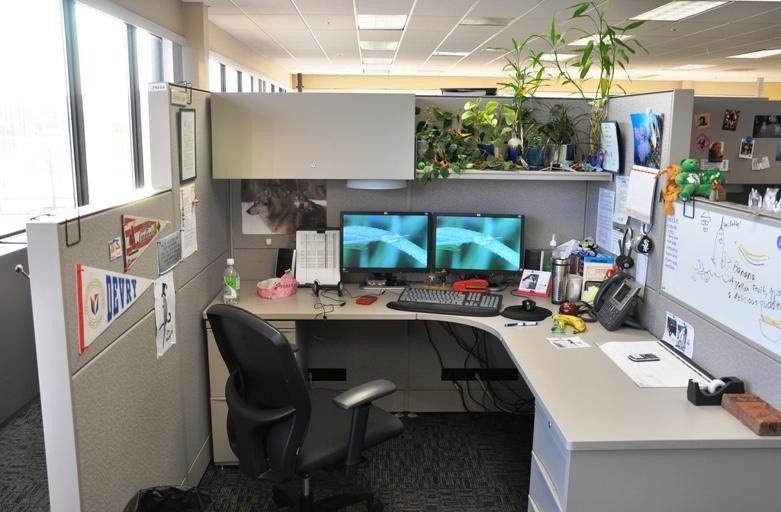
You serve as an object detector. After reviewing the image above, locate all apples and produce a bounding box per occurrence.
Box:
[560,302,576,315]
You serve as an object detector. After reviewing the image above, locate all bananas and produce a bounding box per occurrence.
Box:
[553,314,585,333]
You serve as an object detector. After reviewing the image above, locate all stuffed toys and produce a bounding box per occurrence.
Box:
[659,158,729,218]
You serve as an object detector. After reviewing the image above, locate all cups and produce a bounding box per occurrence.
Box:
[552,257,582,304]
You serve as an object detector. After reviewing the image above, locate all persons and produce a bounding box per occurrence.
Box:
[754,116,781,138]
[709,142,724,161]
[676,327,687,348]
[526,274,536,289]
[725,111,738,131]
[644,120,662,168]
[698,115,709,127]
[743,142,754,157]
[696,136,707,147]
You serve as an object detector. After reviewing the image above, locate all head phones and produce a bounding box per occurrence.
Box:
[616,228,634,269]
[313,280,343,297]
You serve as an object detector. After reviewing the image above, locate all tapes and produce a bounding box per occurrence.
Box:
[707,379,726,394]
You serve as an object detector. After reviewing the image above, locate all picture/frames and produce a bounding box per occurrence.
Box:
[178,108,197,182]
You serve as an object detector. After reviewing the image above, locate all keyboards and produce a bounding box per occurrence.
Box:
[388,287,504,316]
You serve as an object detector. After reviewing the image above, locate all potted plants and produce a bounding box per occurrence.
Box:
[460,99,506,168]
[478,2,649,167]
[414,107,454,166]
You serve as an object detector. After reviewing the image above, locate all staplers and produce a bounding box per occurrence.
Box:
[452,279,490,293]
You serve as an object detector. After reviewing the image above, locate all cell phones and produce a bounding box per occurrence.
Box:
[356,295,377,304]
[629,353,658,362]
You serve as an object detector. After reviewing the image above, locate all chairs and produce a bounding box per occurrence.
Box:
[206,303,404,508]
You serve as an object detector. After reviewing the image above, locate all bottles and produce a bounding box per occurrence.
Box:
[223,258,240,306]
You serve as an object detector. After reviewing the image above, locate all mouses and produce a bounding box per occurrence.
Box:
[523,300,536,311]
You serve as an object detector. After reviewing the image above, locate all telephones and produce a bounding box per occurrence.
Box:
[592,274,640,331]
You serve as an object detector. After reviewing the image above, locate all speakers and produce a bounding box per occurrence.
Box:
[275,247,296,279]
[523,249,543,271]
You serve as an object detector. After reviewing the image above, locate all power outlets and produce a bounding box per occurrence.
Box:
[441,368,518,381]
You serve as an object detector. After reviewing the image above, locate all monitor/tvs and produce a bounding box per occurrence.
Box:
[340,211,433,290]
[432,213,525,291]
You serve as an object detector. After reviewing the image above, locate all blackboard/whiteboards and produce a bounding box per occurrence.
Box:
[659,199,781,363]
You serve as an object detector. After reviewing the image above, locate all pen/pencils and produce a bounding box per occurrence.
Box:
[504,321,537,326]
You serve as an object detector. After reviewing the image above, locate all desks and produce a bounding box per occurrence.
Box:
[204,280,779,512]
